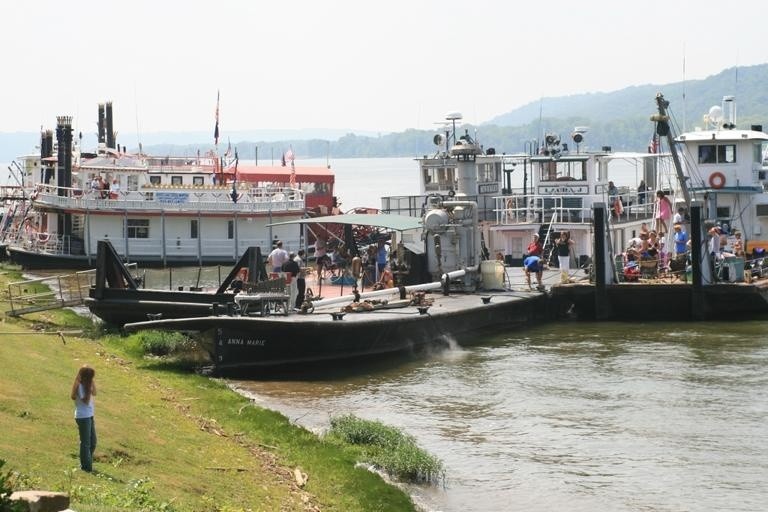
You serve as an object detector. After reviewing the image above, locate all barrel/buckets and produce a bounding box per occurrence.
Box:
[505,254,513,264]
[579,254,589,267]
[480,259,507,292]
[723,256,745,283]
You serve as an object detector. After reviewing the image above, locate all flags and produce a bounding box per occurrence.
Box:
[284,148,293,161]
[226,143,232,158]
[650,122,660,155]
[289,161,296,188]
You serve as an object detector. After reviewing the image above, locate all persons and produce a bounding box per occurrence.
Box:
[556,231,574,281]
[636,181,648,203]
[608,182,623,224]
[655,190,673,234]
[71,365,97,472]
[528,235,544,285]
[266,231,387,308]
[624,208,746,280]
[524,256,547,291]
[72,171,119,201]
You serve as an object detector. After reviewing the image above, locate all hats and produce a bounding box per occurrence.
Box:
[288,251,297,258]
[531,232,541,238]
[673,224,682,230]
[708,225,718,234]
